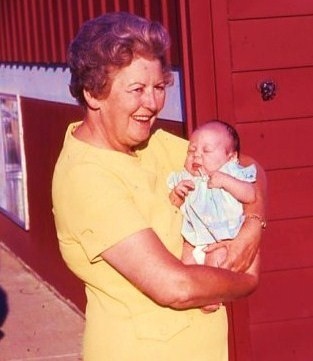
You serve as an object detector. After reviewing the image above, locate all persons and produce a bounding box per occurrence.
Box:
[164,119,257,314]
[51,12,266,361]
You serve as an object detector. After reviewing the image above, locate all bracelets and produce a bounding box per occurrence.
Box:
[241,214,266,234]
